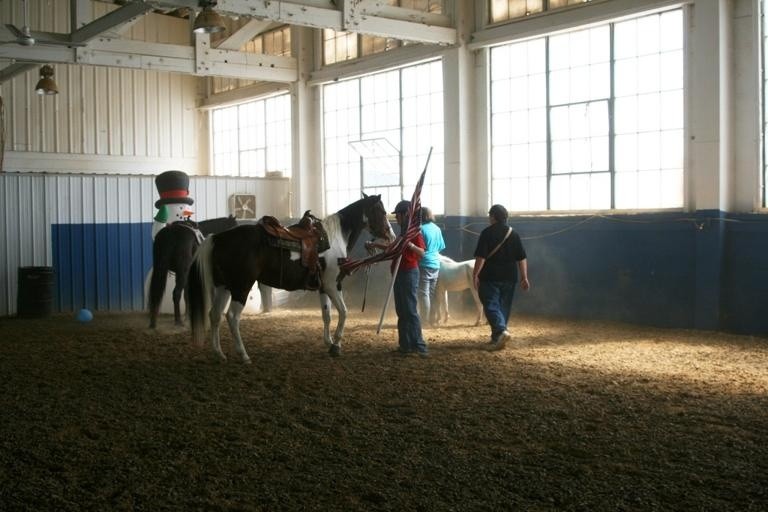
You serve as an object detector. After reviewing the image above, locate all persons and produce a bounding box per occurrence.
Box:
[471,204,532,351]
[364,200,432,357]
[415,206,446,330]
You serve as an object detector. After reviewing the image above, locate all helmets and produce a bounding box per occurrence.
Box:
[391,201,411,214]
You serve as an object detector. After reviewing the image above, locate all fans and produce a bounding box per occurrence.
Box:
[232,194,256,220]
[0,1,88,49]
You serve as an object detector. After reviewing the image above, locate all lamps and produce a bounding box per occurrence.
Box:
[36,65,59,96]
[191,0,226,35]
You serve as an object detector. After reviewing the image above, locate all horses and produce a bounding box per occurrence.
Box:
[435,253,489,326]
[146,213,239,329]
[185,190,397,365]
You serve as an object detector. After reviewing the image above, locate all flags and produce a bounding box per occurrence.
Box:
[337,167,429,276]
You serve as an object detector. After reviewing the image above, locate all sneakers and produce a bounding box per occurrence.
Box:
[486,330,511,350]
[394,349,429,358]
